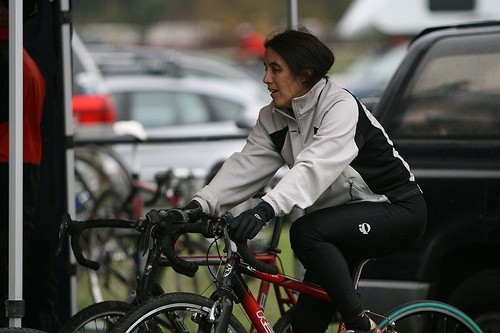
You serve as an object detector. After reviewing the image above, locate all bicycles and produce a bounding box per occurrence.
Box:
[62,135,262,326]
[55,212,480,333]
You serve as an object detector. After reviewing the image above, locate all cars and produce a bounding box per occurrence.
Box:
[86,46,273,195]
[368,19,500,299]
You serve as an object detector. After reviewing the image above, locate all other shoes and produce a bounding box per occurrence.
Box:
[340,310,381,333]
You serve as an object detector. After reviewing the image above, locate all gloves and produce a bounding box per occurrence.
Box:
[146,200,203,223]
[230,200,275,241]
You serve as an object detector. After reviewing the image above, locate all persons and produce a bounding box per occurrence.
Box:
[0,0,45,329]
[163,30,428,333]
